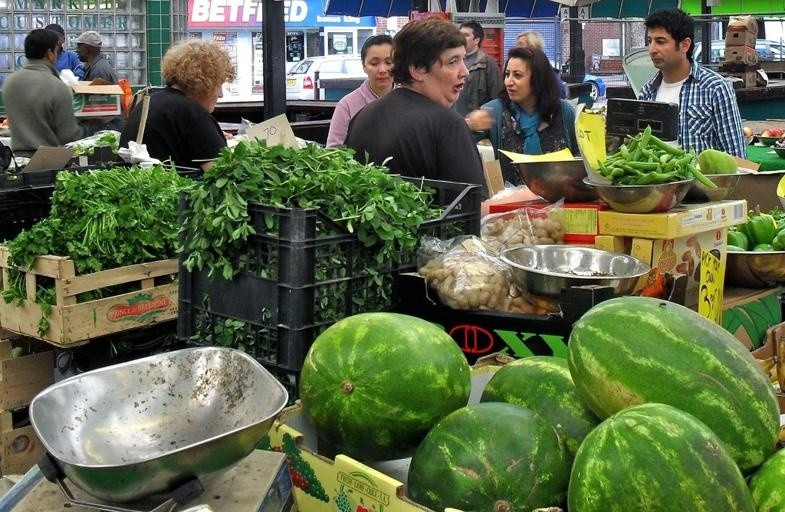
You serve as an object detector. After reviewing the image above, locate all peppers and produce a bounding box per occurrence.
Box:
[592,124,718,190]
[727,203,785,252]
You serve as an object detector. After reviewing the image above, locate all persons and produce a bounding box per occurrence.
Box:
[115,38,235,180]
[344,17,541,218]
[325,34,402,149]
[1,28,91,159]
[514,32,566,100]
[448,19,503,118]
[637,7,746,166]
[70,31,117,85]
[45,23,85,79]
[461,47,580,158]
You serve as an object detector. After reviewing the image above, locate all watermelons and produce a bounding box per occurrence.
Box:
[743,451,785,511]
[566,399,758,511]
[408,402,564,512]
[479,355,596,468]
[300,312,471,458]
[566,295,783,473]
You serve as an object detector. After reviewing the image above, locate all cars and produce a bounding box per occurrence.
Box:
[550,59,606,102]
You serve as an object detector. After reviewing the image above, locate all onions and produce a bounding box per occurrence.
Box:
[743,127,785,143]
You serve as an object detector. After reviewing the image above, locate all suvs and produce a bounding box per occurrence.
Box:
[286,51,368,102]
[689,38,785,63]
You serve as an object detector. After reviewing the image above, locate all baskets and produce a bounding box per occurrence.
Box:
[0,164,200,246]
[177,172,482,372]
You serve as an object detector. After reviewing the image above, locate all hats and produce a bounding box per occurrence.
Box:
[71,30,102,47]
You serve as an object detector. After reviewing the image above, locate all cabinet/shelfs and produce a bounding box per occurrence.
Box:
[0,0,189,116]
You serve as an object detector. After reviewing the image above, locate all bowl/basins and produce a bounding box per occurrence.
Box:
[502,242,656,304]
[725,251,785,289]
[743,134,785,159]
[508,156,751,214]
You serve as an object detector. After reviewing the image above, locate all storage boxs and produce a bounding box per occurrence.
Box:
[716,72,757,87]
[725,30,756,48]
[727,16,761,33]
[70,78,125,123]
[724,45,758,66]
[255,351,515,512]
[686,285,784,352]
